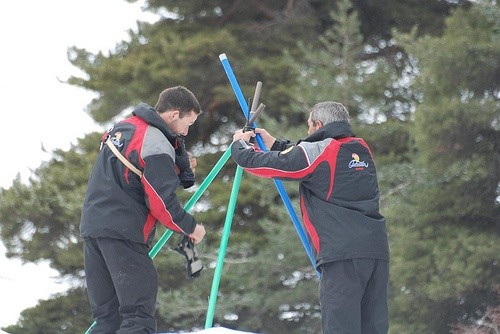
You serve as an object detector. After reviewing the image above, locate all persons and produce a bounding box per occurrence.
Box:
[230,102,389,334]
[80,86,205,334]
